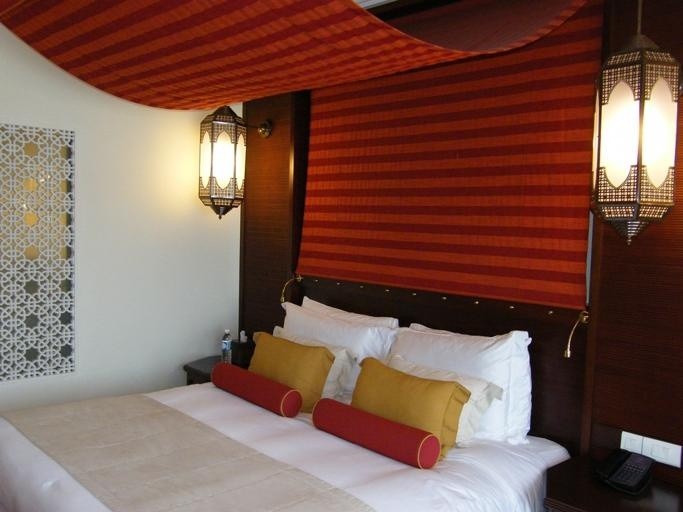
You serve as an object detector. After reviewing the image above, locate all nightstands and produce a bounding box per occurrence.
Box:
[543,449,683,512]
[182,339,255,385]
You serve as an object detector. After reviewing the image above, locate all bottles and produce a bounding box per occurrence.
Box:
[221,329,232,365]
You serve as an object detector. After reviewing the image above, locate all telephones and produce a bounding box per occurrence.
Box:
[599,448,656,492]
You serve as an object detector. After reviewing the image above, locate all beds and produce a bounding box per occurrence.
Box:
[1,276,592,511]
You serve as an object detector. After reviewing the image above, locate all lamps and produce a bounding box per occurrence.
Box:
[592,1,679,245]
[199,105,271,220]
